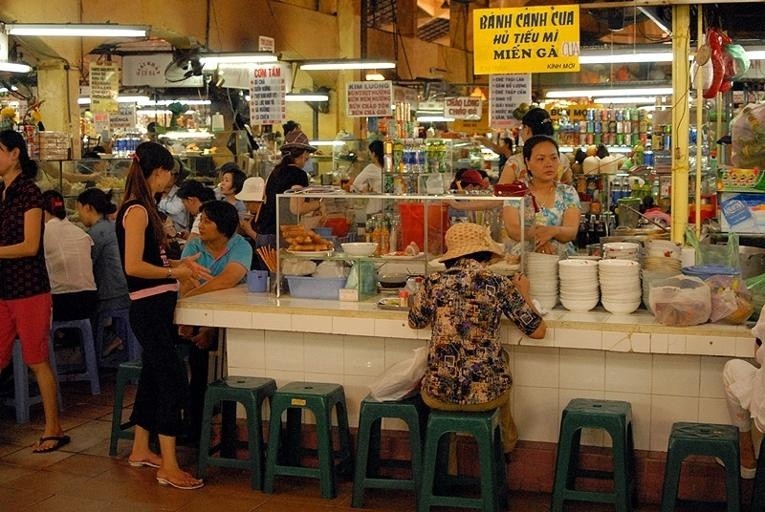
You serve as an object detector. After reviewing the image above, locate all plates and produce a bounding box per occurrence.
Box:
[172,154,181,159]
[96,153,116,159]
[183,151,203,156]
[381,251,425,259]
[596,259,643,315]
[286,248,334,255]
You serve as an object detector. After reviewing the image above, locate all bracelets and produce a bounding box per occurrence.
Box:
[164,267,173,279]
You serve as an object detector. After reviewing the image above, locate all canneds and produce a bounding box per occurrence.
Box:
[384,141,393,193]
[579,108,646,145]
[652,125,672,152]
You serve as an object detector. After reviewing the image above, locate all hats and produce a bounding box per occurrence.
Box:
[461,170,482,184]
[235,177,265,202]
[280,127,317,152]
[438,222,504,264]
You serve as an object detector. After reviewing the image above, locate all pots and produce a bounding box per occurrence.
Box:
[694,232,765,281]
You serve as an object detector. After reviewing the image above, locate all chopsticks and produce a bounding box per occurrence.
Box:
[255,245,276,271]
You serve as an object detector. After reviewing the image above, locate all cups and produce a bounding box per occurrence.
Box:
[643,151,653,165]
[269,271,284,295]
[612,189,629,204]
[246,270,268,291]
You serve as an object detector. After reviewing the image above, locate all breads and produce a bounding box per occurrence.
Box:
[280,223,330,250]
[186,143,199,152]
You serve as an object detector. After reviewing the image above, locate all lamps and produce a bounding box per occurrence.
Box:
[414,109,455,123]
[306,139,347,146]
[0,61,33,74]
[544,0,765,103]
[4,22,152,38]
[297,57,397,79]
[241,90,333,107]
[76,89,215,147]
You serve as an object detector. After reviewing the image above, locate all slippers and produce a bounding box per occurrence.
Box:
[716,456,757,479]
[33,435,71,453]
[157,469,204,490]
[128,454,161,468]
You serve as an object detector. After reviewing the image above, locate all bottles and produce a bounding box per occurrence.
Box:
[412,277,422,307]
[711,156,717,168]
[364,211,403,256]
[381,137,447,196]
[399,290,408,308]
[631,178,651,200]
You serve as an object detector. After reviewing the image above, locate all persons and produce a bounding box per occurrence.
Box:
[710,301,765,480]
[153,159,256,425]
[147,122,164,141]
[0,128,73,454]
[241,121,320,276]
[504,136,581,256]
[350,140,383,194]
[497,108,573,190]
[226,121,258,176]
[115,141,206,489]
[77,187,132,312]
[408,219,546,465]
[39,190,99,361]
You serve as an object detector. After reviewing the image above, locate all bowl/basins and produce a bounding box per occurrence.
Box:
[313,226,332,237]
[600,241,642,259]
[324,218,350,237]
[524,251,558,312]
[556,258,601,312]
[340,242,378,257]
[642,238,683,315]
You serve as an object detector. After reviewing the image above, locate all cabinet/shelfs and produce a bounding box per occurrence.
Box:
[653,108,718,200]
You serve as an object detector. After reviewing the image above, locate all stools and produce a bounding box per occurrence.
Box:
[661,422,741,512]
[0,309,140,455]
[351,400,423,507]
[551,397,637,512]
[198,376,277,490]
[261,381,351,500]
[420,407,505,512]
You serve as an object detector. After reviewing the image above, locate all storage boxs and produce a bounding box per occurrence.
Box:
[719,192,765,234]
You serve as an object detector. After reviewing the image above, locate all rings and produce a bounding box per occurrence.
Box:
[542,250,545,254]
[534,239,540,245]
